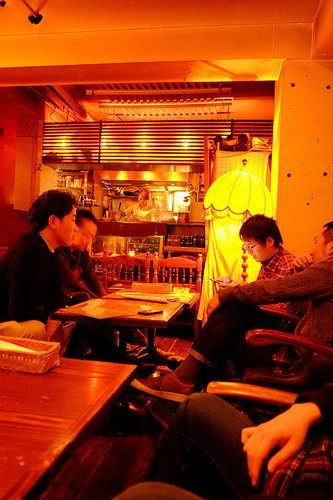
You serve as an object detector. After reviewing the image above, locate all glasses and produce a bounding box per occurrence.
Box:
[244,241,260,251]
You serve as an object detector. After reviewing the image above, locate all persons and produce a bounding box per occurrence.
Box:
[54,208,184,366]
[144,383,333,500]
[129,224,333,402]
[0,189,156,378]
[206,214,308,376]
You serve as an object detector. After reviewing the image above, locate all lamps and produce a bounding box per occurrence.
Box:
[203,160,273,281]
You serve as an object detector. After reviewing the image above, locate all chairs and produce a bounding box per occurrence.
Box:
[1,245,333,416]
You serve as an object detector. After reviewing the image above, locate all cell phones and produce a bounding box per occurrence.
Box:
[138,309,162,315]
[210,278,223,284]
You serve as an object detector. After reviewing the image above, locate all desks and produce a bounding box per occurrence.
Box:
[102,287,200,361]
[51,299,184,364]
[0,357,137,500]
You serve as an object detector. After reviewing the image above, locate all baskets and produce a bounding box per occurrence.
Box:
[0,336,61,374]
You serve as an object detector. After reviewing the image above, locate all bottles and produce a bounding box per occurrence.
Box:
[180,235,205,247]
[104,208,109,218]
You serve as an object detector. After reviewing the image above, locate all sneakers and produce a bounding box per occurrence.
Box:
[130,370,194,403]
